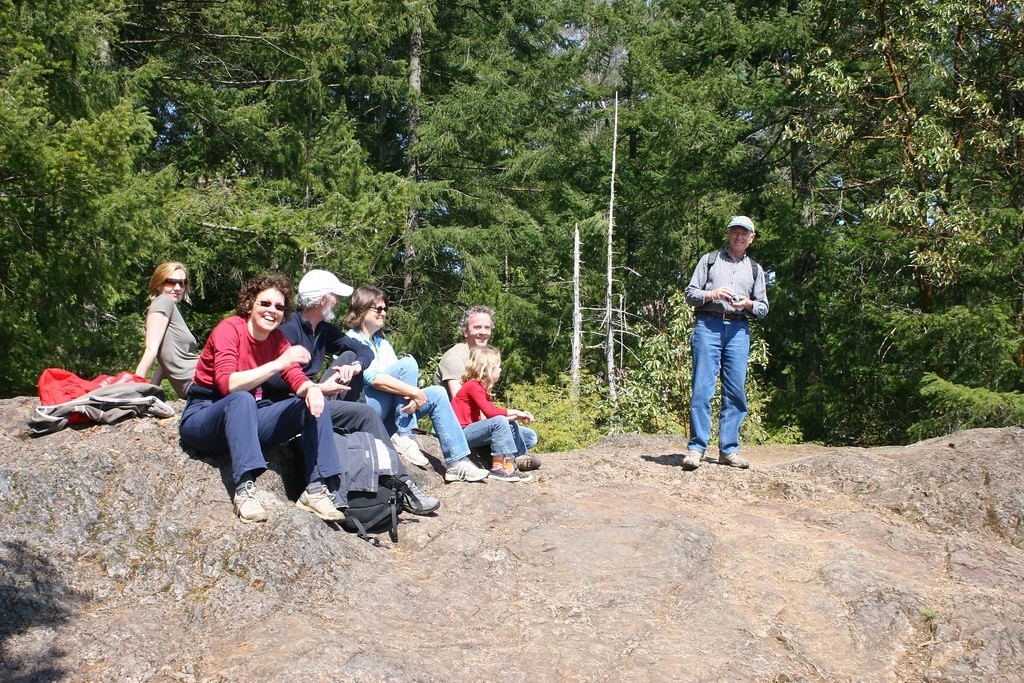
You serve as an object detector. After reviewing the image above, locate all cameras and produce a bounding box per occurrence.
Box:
[728,295,739,302]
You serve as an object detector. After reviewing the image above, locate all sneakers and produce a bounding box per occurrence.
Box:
[682,451,705,468]
[389,433,429,466]
[295,485,345,523]
[444,458,490,482]
[719,452,750,469]
[394,480,441,516]
[233,480,268,525]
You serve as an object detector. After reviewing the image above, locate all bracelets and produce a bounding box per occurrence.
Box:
[305,384,322,392]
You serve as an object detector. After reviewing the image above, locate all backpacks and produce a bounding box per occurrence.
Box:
[322,478,424,547]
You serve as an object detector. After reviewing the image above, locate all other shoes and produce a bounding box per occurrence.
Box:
[488,466,521,483]
[511,469,533,481]
[513,454,542,471]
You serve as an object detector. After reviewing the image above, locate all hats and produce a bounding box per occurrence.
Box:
[727,215,755,233]
[298,269,354,297]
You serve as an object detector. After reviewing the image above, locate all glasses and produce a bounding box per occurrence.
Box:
[366,306,389,313]
[163,278,188,289]
[251,299,286,312]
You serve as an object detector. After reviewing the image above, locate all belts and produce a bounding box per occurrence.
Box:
[696,309,748,321]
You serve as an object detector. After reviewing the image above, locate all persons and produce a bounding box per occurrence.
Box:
[333,284,490,482]
[275,267,441,515]
[450,343,534,482]
[683,215,769,468]
[135,261,202,400]
[432,303,541,471]
[179,273,345,523]
